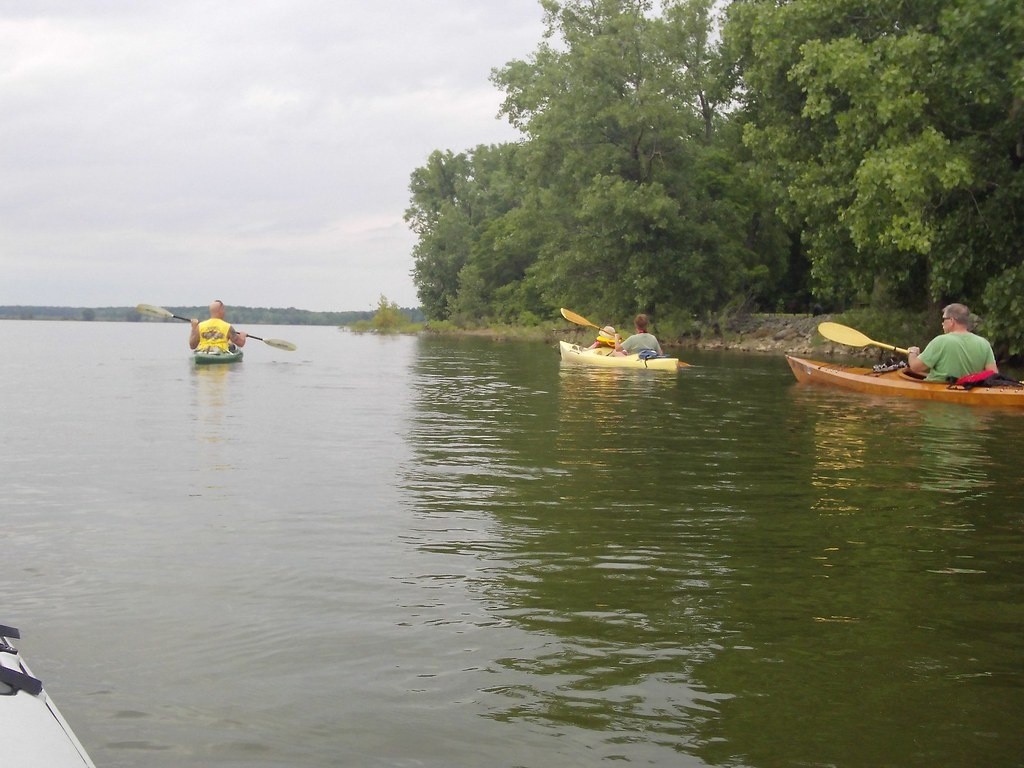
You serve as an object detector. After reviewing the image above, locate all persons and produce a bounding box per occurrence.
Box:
[581,326,622,352]
[906,303,998,384]
[189,300,247,353]
[608,314,663,357]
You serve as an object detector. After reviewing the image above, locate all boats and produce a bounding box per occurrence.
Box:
[784,350,1024,407]
[559,340,679,373]
[194,349,243,365]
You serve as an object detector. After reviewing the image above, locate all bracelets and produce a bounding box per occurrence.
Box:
[907,352,919,356]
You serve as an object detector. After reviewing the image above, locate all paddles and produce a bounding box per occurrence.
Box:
[558,306,692,368]
[816,320,1024,386]
[134,302,298,353]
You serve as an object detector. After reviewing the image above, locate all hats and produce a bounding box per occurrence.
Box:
[599,326,615,339]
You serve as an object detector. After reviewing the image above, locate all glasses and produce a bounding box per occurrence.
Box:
[214,300,223,310]
[942,318,949,322]
